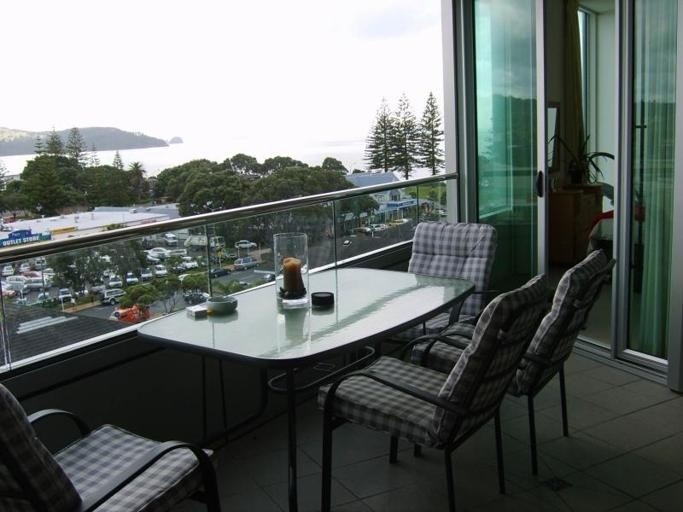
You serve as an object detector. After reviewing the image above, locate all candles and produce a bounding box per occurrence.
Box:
[283,256,304,290]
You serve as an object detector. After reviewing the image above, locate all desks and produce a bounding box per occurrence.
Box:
[137,266,476,512]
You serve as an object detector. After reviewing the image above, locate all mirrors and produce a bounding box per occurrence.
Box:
[546,101,562,173]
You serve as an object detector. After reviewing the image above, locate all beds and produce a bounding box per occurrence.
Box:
[585,218,643,293]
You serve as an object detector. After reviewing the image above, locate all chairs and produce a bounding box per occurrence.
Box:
[416,248,615,472]
[382,221,495,344]
[0,383,220,512]
[320,271,546,510]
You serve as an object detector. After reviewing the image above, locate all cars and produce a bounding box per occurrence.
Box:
[0,232,259,325]
[260,274,275,281]
[349,218,408,234]
[342,240,353,249]
[434,209,448,217]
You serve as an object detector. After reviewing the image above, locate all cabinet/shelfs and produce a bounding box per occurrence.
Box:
[550,187,602,265]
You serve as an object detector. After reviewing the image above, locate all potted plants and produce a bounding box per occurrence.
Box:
[551,134,615,185]
